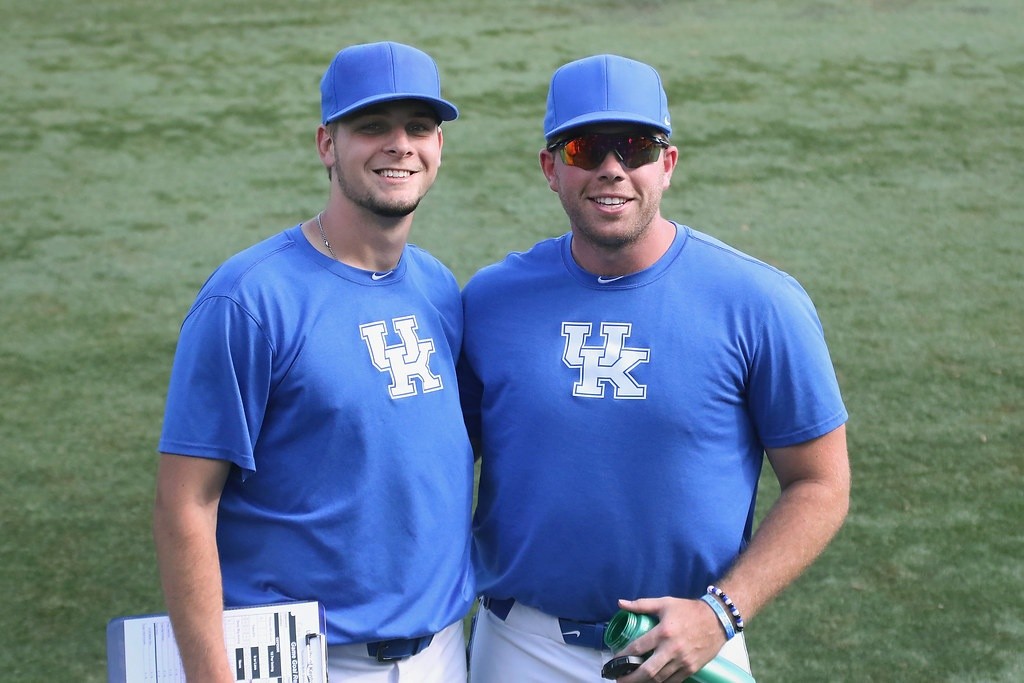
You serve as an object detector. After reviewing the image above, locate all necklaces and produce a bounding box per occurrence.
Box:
[318,212,338,261]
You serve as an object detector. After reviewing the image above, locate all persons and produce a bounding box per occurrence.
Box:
[462,54,851,683]
[153,42,478,683]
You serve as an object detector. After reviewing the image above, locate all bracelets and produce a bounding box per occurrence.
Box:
[700,594,735,641]
[707,586,743,634]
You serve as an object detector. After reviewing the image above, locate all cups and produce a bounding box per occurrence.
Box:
[604,609,756,683]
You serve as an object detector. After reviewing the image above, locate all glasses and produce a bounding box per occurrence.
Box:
[547,131,669,171]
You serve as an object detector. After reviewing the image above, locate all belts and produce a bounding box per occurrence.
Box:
[482,595,611,651]
[367,634,435,662]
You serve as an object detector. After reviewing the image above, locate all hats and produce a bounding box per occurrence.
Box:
[319,42,458,126]
[543,54,672,140]
